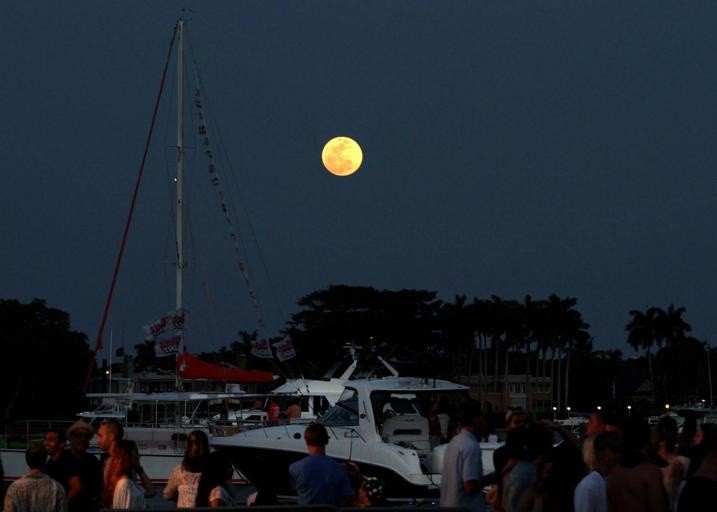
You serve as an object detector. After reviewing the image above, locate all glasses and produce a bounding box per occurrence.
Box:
[70,432,91,438]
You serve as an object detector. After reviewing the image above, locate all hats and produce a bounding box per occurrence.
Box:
[505,407,528,422]
[66,421,93,437]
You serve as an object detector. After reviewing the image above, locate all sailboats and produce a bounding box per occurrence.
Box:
[0,8,303,501]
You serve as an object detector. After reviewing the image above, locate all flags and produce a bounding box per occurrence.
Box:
[162,306,189,331]
[142,312,171,342]
[249,336,274,359]
[270,334,295,362]
[154,331,181,357]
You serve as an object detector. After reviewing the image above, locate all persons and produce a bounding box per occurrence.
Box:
[270,406,290,427]
[290,422,351,511]
[163,429,234,509]
[2,420,156,510]
[424,406,717,510]
